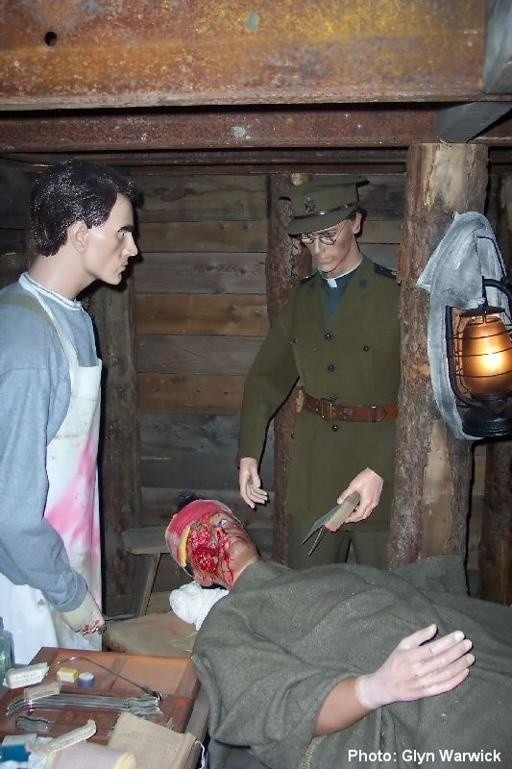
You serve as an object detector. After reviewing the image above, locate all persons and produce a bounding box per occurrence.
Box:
[165,497,512,769]
[237,172,399,566]
[0,162,139,665]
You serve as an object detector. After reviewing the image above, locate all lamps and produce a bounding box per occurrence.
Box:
[445,237,512,436]
